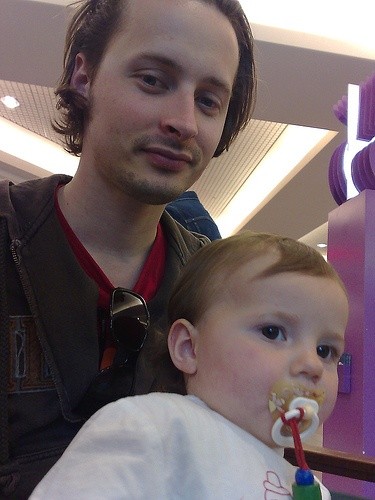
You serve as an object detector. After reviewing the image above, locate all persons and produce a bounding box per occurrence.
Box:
[0,0,257,500]
[27,232,349,500]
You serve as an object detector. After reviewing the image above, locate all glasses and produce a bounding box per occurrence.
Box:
[71,287,150,408]
